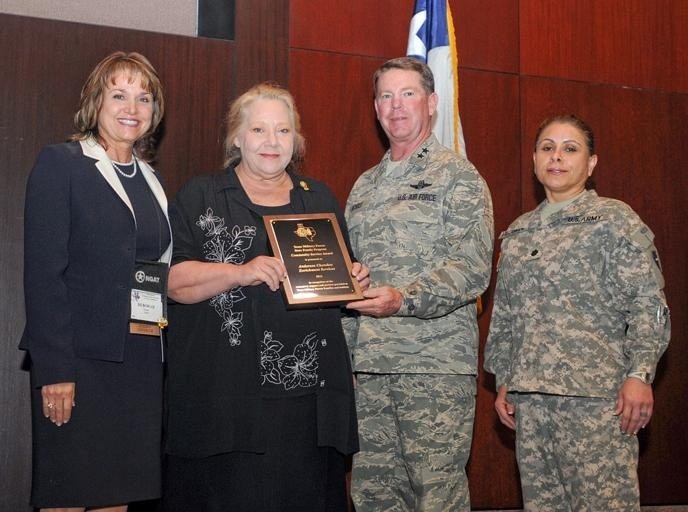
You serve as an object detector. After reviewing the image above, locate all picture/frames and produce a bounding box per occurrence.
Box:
[262,212,365,311]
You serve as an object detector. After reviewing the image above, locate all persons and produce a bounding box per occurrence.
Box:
[343,57,495,510]
[16,51,175,512]
[483,112,672,512]
[163,81,372,511]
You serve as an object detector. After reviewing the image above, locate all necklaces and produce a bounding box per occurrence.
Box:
[110,153,137,179]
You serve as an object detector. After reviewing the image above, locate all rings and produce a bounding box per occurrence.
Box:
[640,412,648,418]
[46,403,56,408]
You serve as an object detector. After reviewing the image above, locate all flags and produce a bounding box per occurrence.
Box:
[405,1,464,162]
